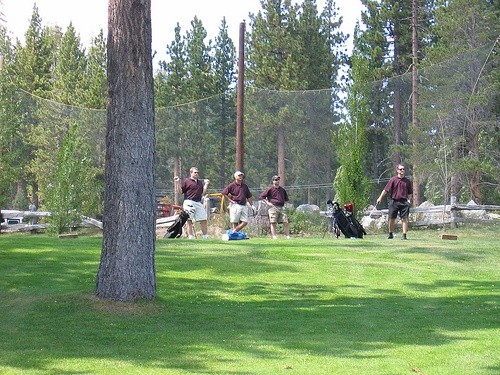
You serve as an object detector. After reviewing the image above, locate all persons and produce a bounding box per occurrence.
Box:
[259,175,290,239]
[174,167,214,239]
[222,171,258,240]
[377,164,413,240]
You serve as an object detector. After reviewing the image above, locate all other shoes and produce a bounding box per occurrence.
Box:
[388,236,393,239]
[273,235,278,239]
[284,235,290,239]
[401,236,406,240]
[203,235,209,239]
[189,235,194,239]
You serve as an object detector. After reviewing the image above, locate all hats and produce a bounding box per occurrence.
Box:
[234,171,244,178]
[272,175,280,181]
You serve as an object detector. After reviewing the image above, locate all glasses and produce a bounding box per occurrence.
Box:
[398,168,405,171]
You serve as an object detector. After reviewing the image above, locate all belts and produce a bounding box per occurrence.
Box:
[192,199,199,202]
